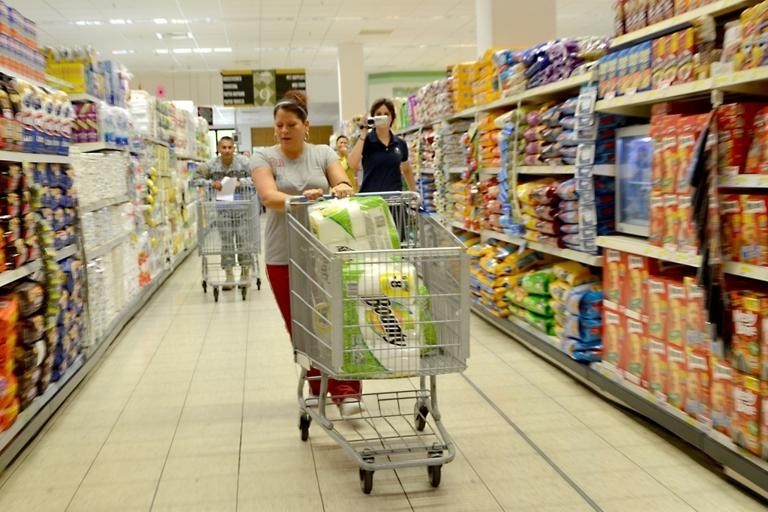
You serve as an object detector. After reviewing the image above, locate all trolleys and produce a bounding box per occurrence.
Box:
[280,191,472,495]
[192,176,265,301]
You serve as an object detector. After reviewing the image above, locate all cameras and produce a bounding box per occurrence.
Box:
[366,113,388,128]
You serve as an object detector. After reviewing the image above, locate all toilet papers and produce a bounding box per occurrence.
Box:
[307,197,436,377]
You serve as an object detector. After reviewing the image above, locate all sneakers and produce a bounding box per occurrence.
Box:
[340,397,365,416]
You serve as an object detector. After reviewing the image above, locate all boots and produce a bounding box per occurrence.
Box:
[223,269,250,290]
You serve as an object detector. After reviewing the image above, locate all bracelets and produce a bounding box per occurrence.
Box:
[359,136,366,141]
[336,182,352,187]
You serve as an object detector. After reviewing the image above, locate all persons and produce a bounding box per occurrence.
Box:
[347,97,417,243]
[190,137,252,290]
[248,91,369,415]
[336,135,359,193]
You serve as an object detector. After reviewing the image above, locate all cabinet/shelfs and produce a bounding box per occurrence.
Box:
[0,67,86,473]
[70,93,141,343]
[594,1,768,502]
[394,71,600,392]
[138,137,212,291]
